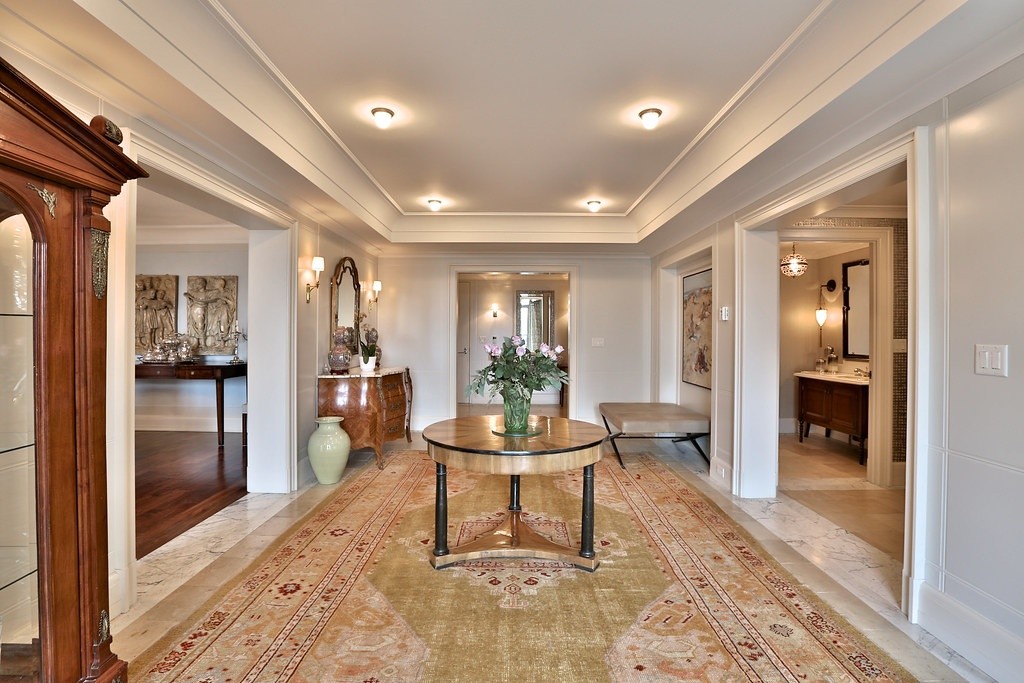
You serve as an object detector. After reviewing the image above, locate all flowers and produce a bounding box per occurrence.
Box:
[465,336,573,429]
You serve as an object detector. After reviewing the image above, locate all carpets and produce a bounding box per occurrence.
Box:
[127,450,923,683]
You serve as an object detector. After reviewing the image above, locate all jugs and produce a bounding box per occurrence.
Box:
[144,332,193,362]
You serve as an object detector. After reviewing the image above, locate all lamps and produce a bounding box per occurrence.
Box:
[780,242,808,279]
[371,108,664,212]
[816,280,837,326]
[492,304,499,317]
[306,257,381,304]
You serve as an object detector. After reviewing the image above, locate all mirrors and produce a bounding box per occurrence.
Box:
[330,257,360,355]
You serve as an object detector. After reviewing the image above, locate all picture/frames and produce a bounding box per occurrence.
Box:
[682,268,712,390]
[841,259,869,359]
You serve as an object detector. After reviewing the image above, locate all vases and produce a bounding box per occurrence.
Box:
[492,387,544,437]
[309,417,352,485]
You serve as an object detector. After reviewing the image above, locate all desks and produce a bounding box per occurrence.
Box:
[317,367,413,470]
[422,414,609,572]
[557,364,569,407]
[599,403,710,469]
[135,361,247,445]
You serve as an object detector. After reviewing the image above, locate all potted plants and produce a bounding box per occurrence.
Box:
[360,341,379,372]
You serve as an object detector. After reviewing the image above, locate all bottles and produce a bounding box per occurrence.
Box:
[365,329,382,369]
[330,328,351,370]
[308,416,351,484]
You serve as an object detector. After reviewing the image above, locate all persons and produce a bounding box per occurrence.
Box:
[136,278,174,350]
[182,278,237,349]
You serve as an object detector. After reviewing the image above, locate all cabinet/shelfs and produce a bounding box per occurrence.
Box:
[0,55,150,683]
[793,376,870,464]
